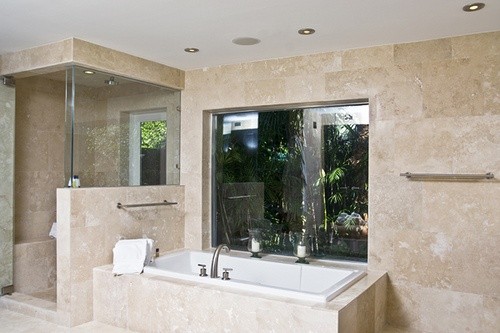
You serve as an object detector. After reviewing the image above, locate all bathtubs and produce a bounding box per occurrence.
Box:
[143,249,367,302]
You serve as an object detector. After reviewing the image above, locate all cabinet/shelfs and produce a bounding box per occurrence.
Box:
[399,172,495,182]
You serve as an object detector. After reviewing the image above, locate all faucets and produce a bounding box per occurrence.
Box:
[211,243,230,278]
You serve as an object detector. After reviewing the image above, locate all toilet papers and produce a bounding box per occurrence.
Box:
[48,223,56,239]
